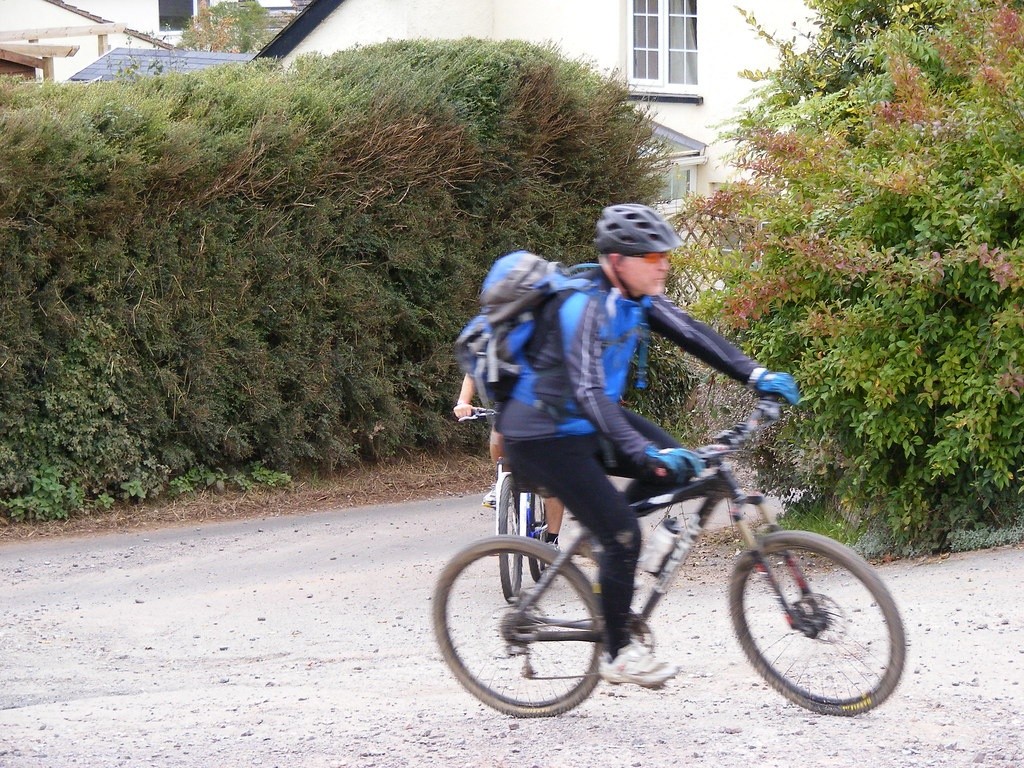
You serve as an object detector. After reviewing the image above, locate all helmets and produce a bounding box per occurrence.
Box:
[593,204,685,256]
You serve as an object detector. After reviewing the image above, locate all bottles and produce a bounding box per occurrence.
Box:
[641,514,680,574]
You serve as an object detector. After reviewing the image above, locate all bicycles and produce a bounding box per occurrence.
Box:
[457,405,547,604]
[431,394,906,719]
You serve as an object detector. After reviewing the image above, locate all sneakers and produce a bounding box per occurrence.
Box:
[483,483,498,509]
[597,639,680,688]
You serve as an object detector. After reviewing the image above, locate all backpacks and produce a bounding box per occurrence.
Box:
[456,246,603,402]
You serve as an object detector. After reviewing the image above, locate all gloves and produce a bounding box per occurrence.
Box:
[636,441,703,490]
[748,366,801,406]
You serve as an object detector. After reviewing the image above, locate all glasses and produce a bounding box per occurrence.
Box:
[632,251,670,263]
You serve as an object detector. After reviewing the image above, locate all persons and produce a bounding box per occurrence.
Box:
[450,372,564,554]
[466,207,802,686]
